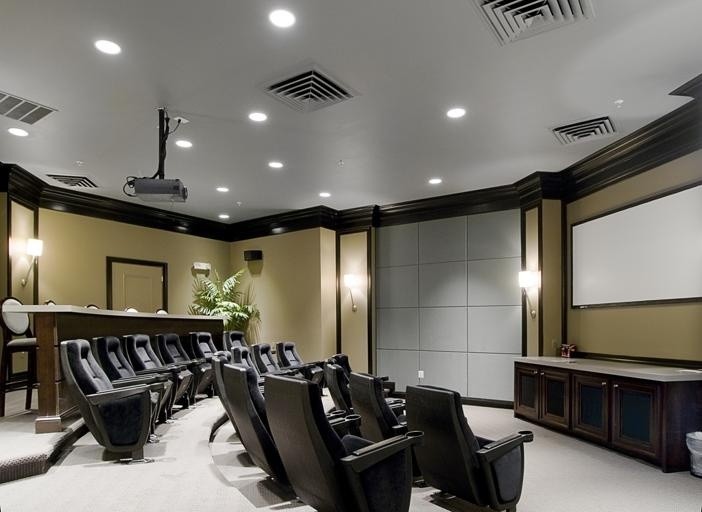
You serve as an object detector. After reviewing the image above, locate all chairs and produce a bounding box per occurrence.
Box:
[0,297,36,417]
[210,332,535,512]
[59,329,251,465]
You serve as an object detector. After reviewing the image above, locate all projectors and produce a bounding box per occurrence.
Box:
[134,178,188,203]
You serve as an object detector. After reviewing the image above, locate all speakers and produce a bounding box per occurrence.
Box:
[244,249,262,261]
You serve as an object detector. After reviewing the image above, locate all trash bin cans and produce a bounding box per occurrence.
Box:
[686,431,702,478]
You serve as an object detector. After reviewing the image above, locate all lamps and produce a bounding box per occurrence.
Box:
[21,239,42,287]
[343,274,357,311]
[519,272,537,318]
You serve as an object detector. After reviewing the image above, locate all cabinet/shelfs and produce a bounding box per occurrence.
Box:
[514,361,702,473]
